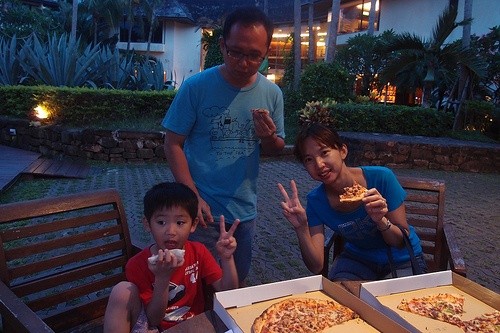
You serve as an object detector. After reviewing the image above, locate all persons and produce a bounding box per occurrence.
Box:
[103,182,241,333]
[160,7,286,333]
[277,125,426,281]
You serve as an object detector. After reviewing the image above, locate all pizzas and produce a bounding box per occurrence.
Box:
[251,297,359,333]
[149,248,185,265]
[339,183,369,202]
[397,293,464,328]
[250,108,270,114]
[461,311,500,333]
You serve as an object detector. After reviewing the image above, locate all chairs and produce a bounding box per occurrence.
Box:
[320,176,468,282]
[0,189,143,333]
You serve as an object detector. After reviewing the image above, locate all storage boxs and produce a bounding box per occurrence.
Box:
[359,269,500,333]
[212,274,413,333]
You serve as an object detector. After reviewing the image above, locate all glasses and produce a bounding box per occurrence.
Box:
[224,40,267,64]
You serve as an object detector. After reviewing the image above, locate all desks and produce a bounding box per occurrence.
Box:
[161,280,375,333]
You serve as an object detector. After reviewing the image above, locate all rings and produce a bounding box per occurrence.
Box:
[383,201,385,207]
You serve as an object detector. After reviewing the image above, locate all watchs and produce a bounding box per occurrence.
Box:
[376,220,392,232]
